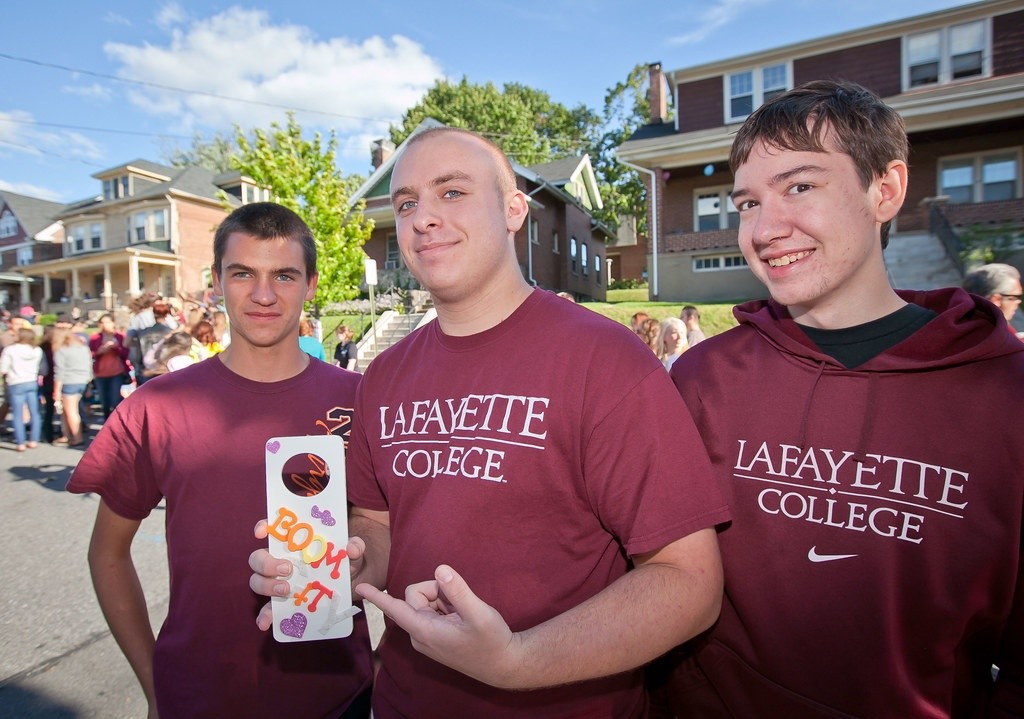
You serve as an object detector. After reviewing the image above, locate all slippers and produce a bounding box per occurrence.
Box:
[65,440,87,450]
[52,438,71,447]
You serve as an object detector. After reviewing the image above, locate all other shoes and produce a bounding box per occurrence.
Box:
[17,442,37,451]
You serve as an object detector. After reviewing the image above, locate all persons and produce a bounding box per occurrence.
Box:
[0,290,231,452]
[627,305,706,376]
[67,202,374,719]
[555,291,573,302]
[298,315,325,362]
[248,128,735,719]
[966,263,1022,339]
[330,325,358,374]
[669,80,1024,719]
